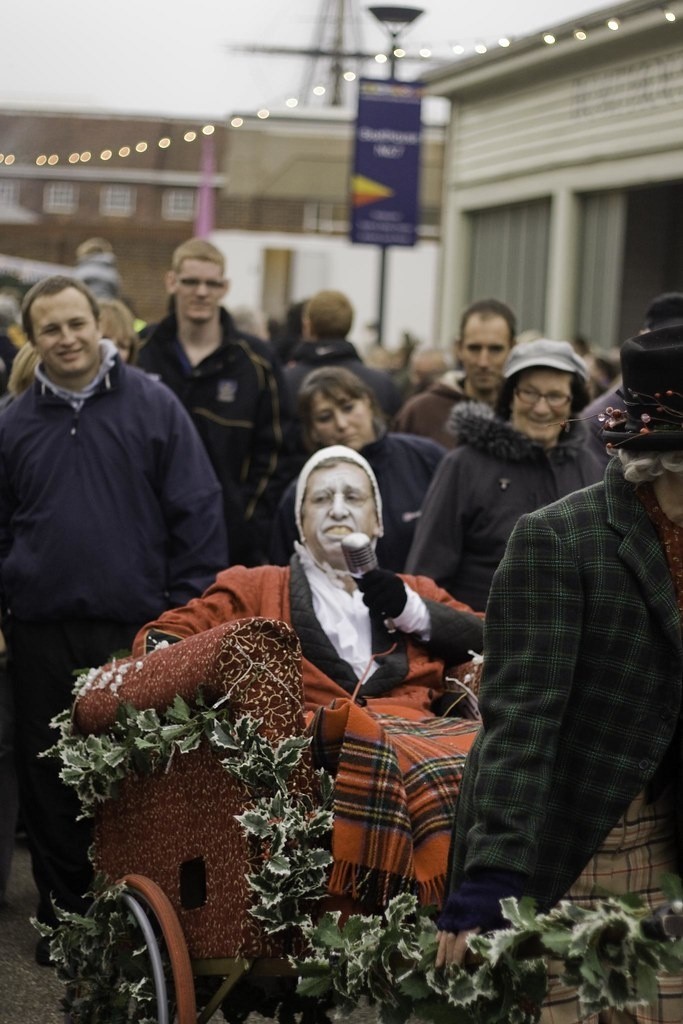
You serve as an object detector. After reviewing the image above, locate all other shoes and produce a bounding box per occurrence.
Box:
[34,922,65,966]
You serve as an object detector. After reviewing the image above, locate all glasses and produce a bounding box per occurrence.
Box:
[169,275,225,289]
[511,384,576,409]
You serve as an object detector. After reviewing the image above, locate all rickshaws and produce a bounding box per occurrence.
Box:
[59,614,683,1024]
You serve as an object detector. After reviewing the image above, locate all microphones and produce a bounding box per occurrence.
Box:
[339,532,398,642]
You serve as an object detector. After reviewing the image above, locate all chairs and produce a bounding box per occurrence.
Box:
[75,616,485,956]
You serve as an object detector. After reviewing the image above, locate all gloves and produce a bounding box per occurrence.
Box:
[358,568,407,620]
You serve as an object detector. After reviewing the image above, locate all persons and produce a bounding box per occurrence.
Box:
[437,321,683,1024]
[262,368,448,578]
[0,237,683,576]
[402,340,612,625]
[136,444,489,921]
[0,278,223,967]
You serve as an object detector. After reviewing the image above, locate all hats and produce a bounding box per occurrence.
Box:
[502,339,587,388]
[293,445,383,589]
[646,292,683,328]
[579,325,683,450]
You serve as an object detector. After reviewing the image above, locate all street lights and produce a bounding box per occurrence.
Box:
[360,4,428,356]
